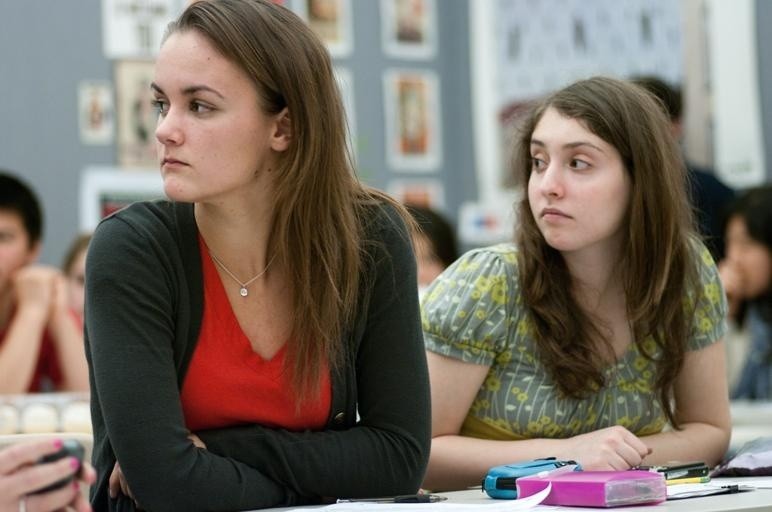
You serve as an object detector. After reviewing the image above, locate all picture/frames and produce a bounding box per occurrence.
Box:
[389,178,444,214]
[384,69,444,172]
[293,2,356,170]
[75,1,187,235]
[381,2,437,62]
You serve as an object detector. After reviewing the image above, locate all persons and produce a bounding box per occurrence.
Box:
[407,206,456,291]
[83,2,431,512]
[64,234,94,327]
[1,168,90,396]
[0,438,98,512]
[419,76,731,491]
[626,78,733,265]
[717,182,772,403]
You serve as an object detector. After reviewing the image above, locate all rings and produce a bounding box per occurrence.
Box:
[19,498,26,512]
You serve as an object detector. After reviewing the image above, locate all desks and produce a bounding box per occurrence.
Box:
[255,403,772,511]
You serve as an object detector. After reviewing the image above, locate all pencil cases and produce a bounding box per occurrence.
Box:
[516,470,668,507]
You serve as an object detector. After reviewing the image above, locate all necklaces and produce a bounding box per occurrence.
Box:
[210,249,281,297]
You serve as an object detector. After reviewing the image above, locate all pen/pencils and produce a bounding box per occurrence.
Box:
[335,494,449,505]
[666,476,711,486]
